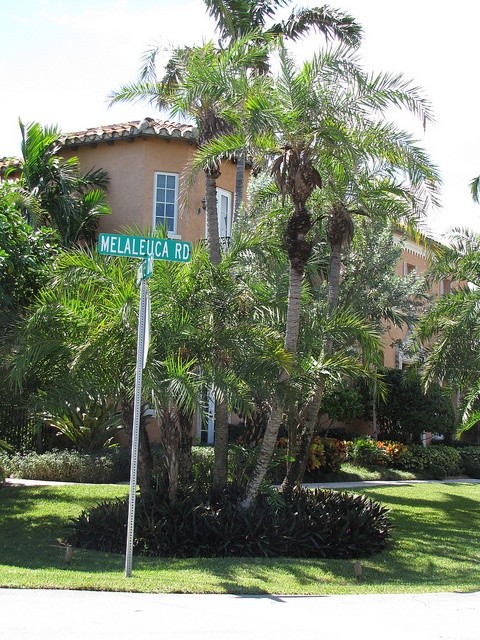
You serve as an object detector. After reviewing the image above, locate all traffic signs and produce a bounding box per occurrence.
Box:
[135,252,155,288]
[97,232,193,263]
